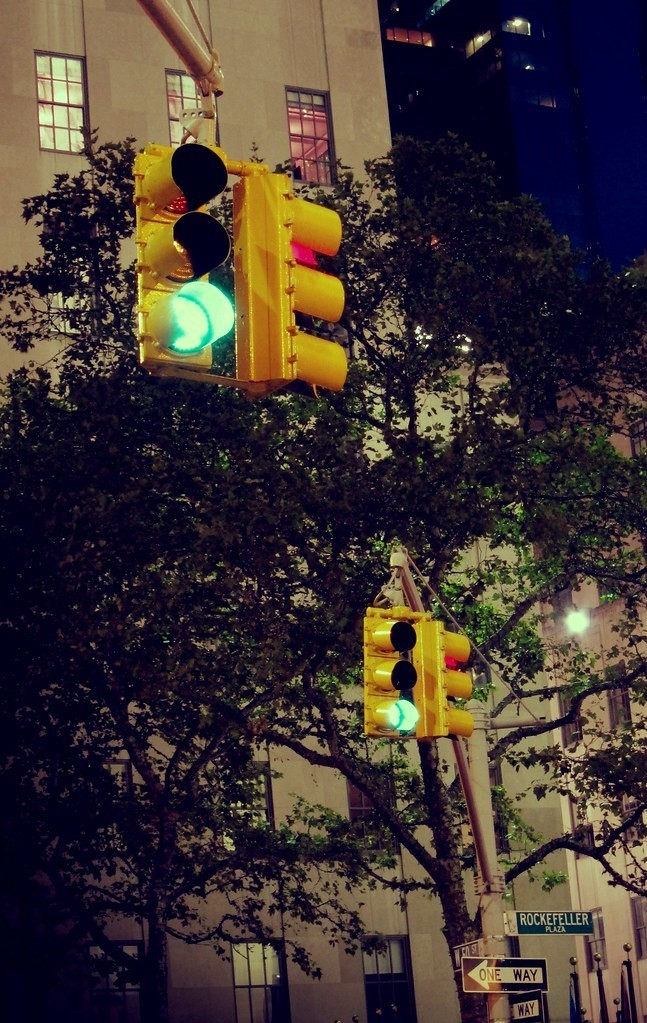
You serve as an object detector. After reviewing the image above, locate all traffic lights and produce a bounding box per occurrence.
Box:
[132,141,235,374]
[410,621,474,740]
[230,172,346,398]
[361,606,419,739]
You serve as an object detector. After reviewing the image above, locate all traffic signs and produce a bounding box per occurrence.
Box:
[486,989,544,1023]
[461,956,549,994]
